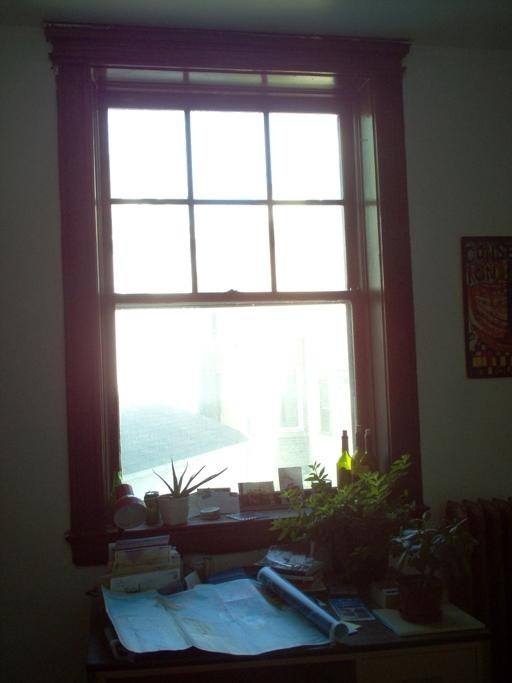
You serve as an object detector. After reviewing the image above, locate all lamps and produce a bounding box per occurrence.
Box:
[113,480,148,542]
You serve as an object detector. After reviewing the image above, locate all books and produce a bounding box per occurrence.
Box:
[369,602,486,638]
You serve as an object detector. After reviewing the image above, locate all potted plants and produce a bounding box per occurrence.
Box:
[156,462,215,526]
[269,453,481,622]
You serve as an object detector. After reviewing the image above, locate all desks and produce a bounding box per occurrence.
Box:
[82,571,491,683]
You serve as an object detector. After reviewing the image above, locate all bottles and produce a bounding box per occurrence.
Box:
[336,429,352,489]
[351,424,379,484]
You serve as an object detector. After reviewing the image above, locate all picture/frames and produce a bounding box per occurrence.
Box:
[457,232,512,378]
